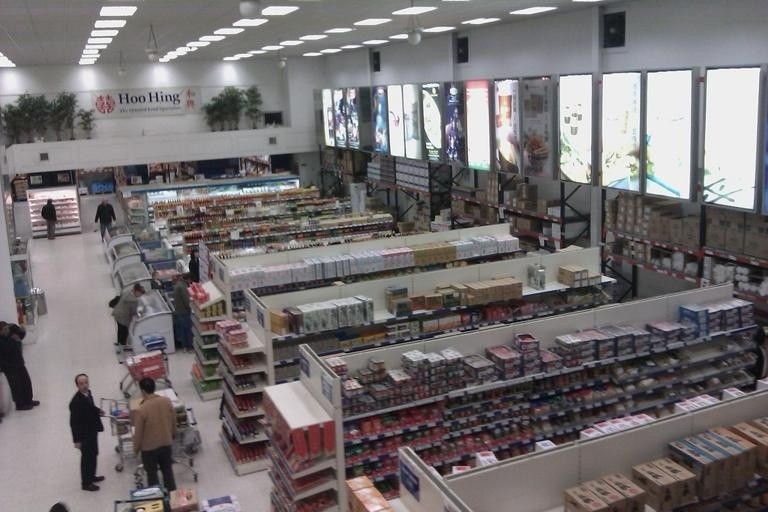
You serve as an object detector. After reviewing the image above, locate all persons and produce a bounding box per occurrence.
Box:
[95,198,116,243]
[173,274,195,354]
[189,248,199,282]
[42,199,57,239]
[69,374,105,491]
[110,283,145,345]
[151,271,171,292]
[133,377,177,491]
[49,502,70,512]
[0,321,40,410]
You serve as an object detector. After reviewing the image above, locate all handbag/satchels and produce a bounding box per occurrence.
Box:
[109,296,120,308]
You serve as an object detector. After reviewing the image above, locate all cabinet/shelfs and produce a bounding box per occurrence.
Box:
[231,247,529,316]
[451,195,591,255]
[343,325,760,498]
[600,225,703,300]
[320,148,397,208]
[390,157,450,221]
[271,272,620,383]
[260,380,337,512]
[700,244,768,309]
[27,187,82,240]
[150,186,399,259]
[11,239,36,332]
[122,199,148,235]
[214,321,269,476]
[188,282,226,401]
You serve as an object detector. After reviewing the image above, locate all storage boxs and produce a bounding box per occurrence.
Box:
[603,191,700,276]
[169,485,199,512]
[130,484,164,504]
[453,171,561,233]
[126,350,167,381]
[564,416,767,511]
[128,388,183,426]
[133,500,164,512]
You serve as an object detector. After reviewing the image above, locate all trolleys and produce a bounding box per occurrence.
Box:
[114,343,177,399]
[99,398,199,483]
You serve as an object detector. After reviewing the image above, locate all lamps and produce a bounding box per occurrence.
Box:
[116,50,125,76]
[405,0,421,47]
[276,49,285,70]
[145,24,160,64]
[239,0,260,17]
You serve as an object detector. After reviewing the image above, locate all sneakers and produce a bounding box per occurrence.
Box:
[16,405,33,410]
[83,484,100,491]
[93,476,105,482]
[30,401,40,406]
